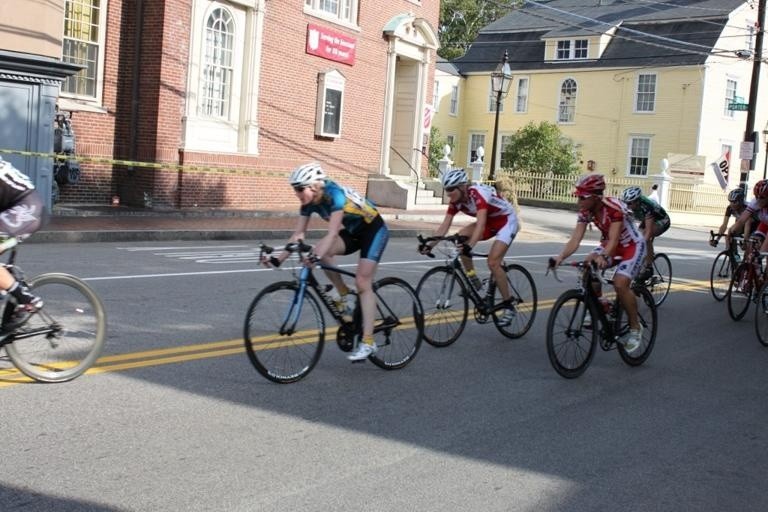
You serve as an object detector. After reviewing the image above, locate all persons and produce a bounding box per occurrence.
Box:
[549,174,648,353]
[725,179,768,313]
[261,162,390,361]
[709,188,760,269]
[419,168,520,327]
[0,156,45,332]
[623,186,671,296]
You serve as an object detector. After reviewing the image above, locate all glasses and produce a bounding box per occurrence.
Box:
[445,186,457,192]
[579,194,602,200]
[295,187,307,192]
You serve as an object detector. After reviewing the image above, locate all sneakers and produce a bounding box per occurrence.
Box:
[638,266,653,281]
[342,286,359,315]
[497,304,518,327]
[624,322,644,353]
[458,278,482,296]
[2,295,44,333]
[346,341,380,361]
[582,311,592,327]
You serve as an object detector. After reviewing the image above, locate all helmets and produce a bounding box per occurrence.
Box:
[728,188,744,202]
[289,161,326,189]
[572,173,606,196]
[443,167,468,188]
[754,179,768,197]
[621,185,641,203]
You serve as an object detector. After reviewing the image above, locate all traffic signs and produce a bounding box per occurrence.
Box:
[728,102,748,111]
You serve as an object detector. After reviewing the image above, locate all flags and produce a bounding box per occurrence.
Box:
[712,147,731,190]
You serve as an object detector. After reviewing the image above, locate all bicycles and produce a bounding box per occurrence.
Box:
[708,226,767,350]
[412,232,538,350]
[541,257,659,378]
[599,253,672,313]
[0,233,106,385]
[244,238,426,385]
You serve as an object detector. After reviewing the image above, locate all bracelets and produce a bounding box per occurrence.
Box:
[600,253,609,262]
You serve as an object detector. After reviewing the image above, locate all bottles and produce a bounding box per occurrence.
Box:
[598,296,609,315]
[325,284,345,315]
[464,267,482,291]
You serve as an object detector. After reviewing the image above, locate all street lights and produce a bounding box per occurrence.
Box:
[487,50,516,182]
